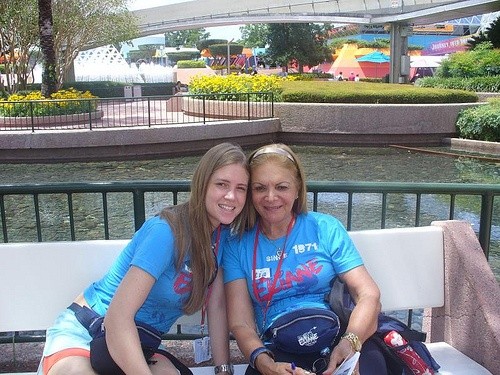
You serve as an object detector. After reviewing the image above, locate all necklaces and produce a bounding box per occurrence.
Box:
[261,224,285,256]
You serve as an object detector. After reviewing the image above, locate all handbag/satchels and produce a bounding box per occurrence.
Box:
[67,302,162,375]
[268,308,340,355]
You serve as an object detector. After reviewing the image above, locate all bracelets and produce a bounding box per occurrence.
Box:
[249,347,276,369]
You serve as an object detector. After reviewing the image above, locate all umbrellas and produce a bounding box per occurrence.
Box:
[356,49,390,79]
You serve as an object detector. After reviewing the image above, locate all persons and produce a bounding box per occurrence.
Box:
[35,141,251,375]
[251,64,258,75]
[337,71,359,82]
[175,81,181,94]
[223,142,382,375]
[278,67,288,77]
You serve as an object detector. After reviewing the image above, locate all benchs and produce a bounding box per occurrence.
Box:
[0,221,500,375]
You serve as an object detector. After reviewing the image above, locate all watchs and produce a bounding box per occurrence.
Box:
[214,362,235,375]
[340,331,362,352]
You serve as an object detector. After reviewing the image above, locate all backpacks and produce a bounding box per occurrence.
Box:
[323,276,441,375]
